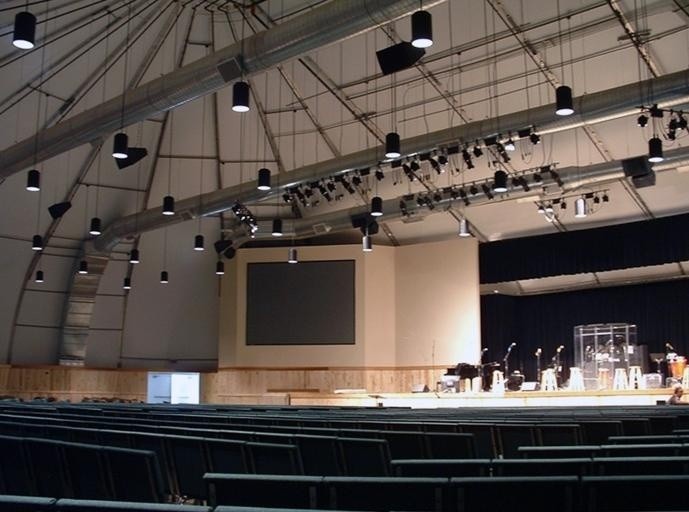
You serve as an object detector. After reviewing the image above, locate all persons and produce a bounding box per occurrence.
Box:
[668,388,684,404]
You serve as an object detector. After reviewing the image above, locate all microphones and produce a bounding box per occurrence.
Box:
[666,343,673,350]
[557,345,565,352]
[509,342,517,347]
[482,348,488,351]
[535,348,542,356]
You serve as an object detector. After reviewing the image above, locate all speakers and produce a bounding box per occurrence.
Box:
[622,156,655,188]
[214,240,236,259]
[376,41,426,75]
[116,148,147,170]
[351,212,378,235]
[48,202,72,220]
